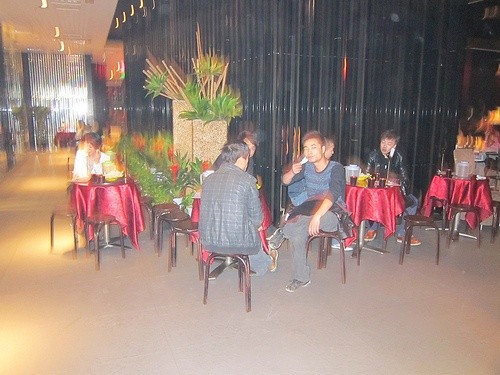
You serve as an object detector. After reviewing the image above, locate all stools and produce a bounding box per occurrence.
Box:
[399,215,440,266]
[202,252,252,312]
[306,230,346,286]
[447,204,480,247]
[140,196,205,281]
[50,204,79,252]
[83,214,126,271]
[489,200,500,243]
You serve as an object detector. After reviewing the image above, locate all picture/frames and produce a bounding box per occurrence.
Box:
[173,197,181,204]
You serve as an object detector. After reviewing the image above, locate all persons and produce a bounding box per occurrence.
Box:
[71,134,111,180]
[478,116,500,153]
[73,119,91,140]
[363,130,422,245]
[197,129,279,276]
[268,129,353,292]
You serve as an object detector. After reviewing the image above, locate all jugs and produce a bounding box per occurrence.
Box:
[455,161,474,180]
[199,170,215,185]
[343,164,361,185]
[102,161,119,182]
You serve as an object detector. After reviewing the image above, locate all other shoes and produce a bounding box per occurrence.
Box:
[331,242,355,251]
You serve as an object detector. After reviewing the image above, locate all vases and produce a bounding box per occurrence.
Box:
[186,205,193,216]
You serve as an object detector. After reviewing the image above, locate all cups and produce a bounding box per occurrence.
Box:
[98,176,105,184]
[368,178,375,188]
[446,169,452,178]
[379,178,386,187]
[350,176,357,186]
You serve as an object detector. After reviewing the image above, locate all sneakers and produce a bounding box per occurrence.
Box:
[269,228,286,249]
[364,230,376,241]
[285,279,311,292]
[268,249,278,273]
[397,237,421,246]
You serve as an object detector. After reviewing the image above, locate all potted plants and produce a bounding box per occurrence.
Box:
[142,21,245,193]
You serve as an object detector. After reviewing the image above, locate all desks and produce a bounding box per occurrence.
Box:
[69,177,147,253]
[420,172,493,243]
[190,190,273,281]
[54,131,75,147]
[345,184,403,257]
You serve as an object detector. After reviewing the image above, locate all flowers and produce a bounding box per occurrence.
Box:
[115,130,214,210]
